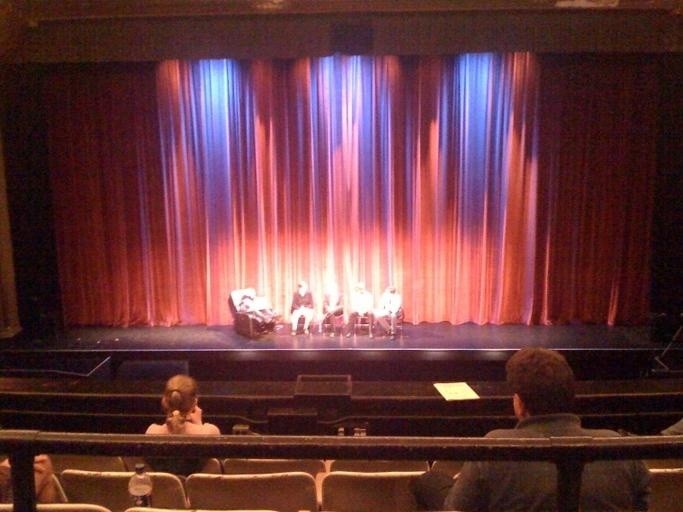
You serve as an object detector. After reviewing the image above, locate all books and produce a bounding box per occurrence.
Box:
[430,380,482,402]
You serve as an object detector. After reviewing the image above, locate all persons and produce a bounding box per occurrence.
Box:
[316,283,345,338]
[344,281,376,340]
[616,417,682,451]
[140,373,222,437]
[0,452,69,506]
[239,290,284,325]
[288,280,314,335]
[372,286,404,342]
[404,344,654,511]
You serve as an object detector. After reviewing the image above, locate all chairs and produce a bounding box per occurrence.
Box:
[229,287,275,338]
[0,453,683,511]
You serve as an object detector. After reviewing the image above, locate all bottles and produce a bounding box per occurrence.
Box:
[337,428,344,436]
[354,428,366,436]
[128,464,152,507]
